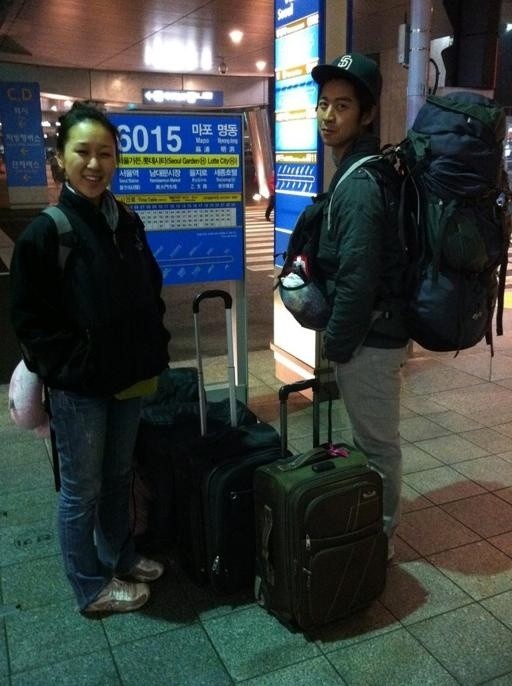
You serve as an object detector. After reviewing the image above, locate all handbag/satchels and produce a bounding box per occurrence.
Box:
[278,195,326,327]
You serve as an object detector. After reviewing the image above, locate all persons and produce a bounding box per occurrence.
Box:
[265,171,275,222]
[307,49,411,564]
[9,100,173,618]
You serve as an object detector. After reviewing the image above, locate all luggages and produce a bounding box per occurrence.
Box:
[255,379,388,634]
[180,288,293,593]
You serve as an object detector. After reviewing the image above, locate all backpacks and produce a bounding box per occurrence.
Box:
[327,93,505,351]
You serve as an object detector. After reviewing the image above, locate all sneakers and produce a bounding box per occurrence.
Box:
[82,577,150,612]
[125,560,163,583]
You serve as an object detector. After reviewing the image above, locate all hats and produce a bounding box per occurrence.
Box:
[311,51,382,93]
